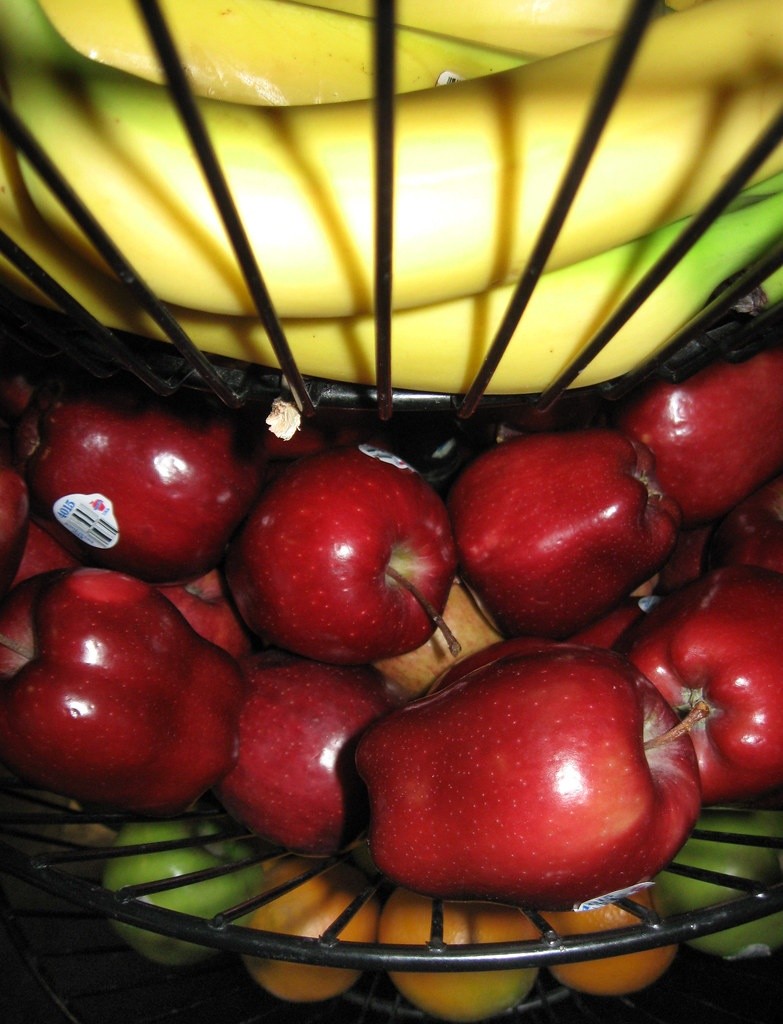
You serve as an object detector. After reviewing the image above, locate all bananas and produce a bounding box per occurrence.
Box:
[0,0,783,397]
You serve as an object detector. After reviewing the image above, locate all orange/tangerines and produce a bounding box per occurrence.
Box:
[236,852,675,1020]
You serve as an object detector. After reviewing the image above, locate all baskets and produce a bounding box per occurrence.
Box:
[1,0,783,1024]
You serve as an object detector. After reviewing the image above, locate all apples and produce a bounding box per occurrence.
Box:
[652,799,783,956]
[99,817,264,969]
[0,362,783,903]
